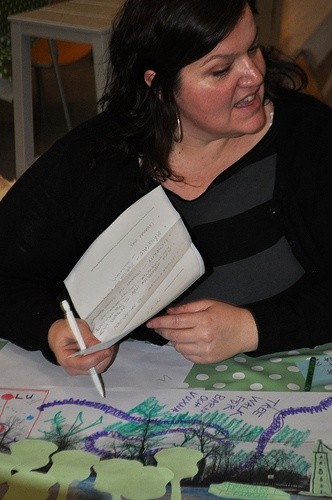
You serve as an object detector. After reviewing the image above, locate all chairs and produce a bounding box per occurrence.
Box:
[32,39,93,132]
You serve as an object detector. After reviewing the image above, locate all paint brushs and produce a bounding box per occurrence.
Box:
[61,300,106,401]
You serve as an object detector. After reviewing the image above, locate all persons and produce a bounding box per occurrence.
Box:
[0,0,332,377]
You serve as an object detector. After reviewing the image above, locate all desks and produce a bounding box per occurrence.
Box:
[7,1,127,182]
